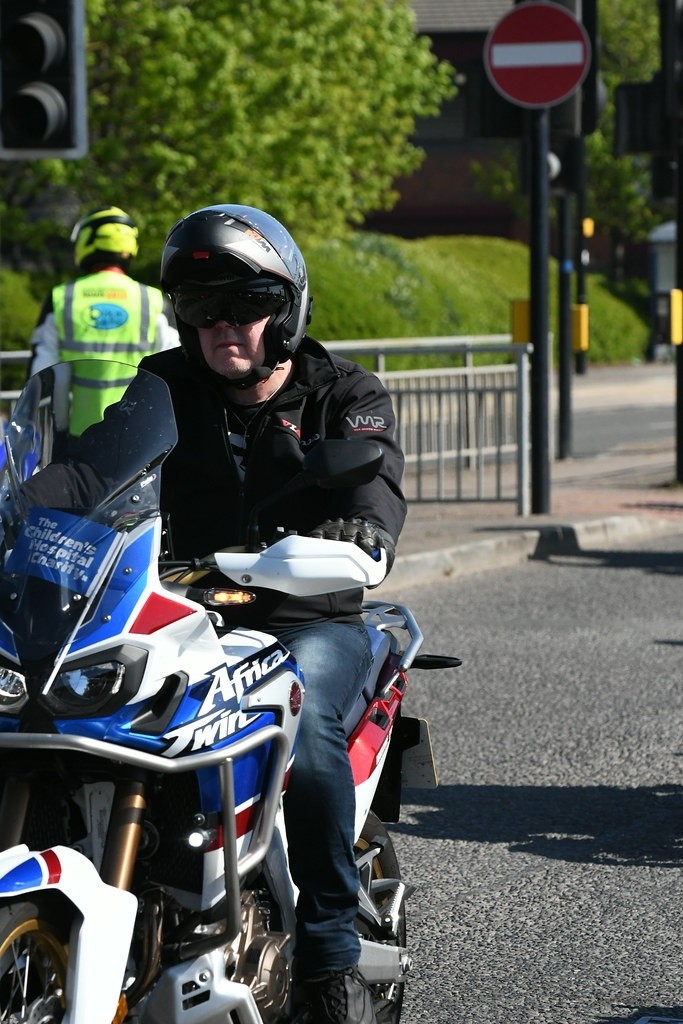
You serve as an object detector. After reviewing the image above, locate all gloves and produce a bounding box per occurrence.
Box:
[310,518,387,590]
[0,503,14,560]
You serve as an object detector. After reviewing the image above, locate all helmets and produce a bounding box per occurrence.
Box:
[70,205,139,273]
[160,204,310,365]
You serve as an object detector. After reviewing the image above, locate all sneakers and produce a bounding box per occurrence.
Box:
[289,955,376,1024]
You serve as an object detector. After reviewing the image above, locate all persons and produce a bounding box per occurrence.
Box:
[0,205,407,1024]
[27,207,182,466]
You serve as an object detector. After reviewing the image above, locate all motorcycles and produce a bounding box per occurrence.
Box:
[0,355,464,1023]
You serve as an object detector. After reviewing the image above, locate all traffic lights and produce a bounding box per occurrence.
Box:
[0,2,93,159]
[516,137,584,194]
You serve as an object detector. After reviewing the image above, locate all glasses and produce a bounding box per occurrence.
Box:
[174,283,293,330]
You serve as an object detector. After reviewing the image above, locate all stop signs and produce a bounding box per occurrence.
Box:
[480,0,592,113]
